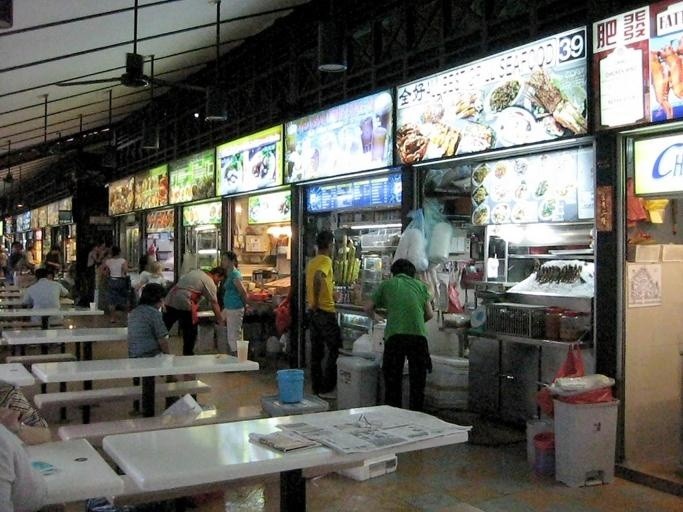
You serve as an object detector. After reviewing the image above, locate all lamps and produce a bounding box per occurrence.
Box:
[97,89,117,171]
[16,167,23,209]
[140,55,162,150]
[205,1,227,123]
[317,2,348,74]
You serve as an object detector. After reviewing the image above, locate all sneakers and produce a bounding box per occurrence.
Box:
[317,389,337,403]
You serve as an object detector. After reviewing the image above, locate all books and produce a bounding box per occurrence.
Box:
[248,409,472,454]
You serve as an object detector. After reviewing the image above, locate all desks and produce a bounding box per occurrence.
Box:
[0,285,19,292]
[0,326,128,359]
[0,297,74,310]
[31,352,261,416]
[1,306,105,330]
[1,292,22,298]
[103,403,469,512]
[25,437,125,510]
[2,362,36,388]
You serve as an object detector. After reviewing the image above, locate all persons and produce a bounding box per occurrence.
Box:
[102,246,128,324]
[88,243,104,302]
[162,268,225,356]
[45,247,61,279]
[134,263,167,292]
[363,258,434,411]
[305,232,341,394]
[1,245,38,283]
[23,269,69,323]
[224,253,249,356]
[0,424,41,512]
[0,380,50,444]
[128,283,171,358]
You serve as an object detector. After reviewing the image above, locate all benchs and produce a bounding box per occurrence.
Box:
[104,462,365,508]
[34,379,212,410]
[58,406,273,447]
[7,351,77,365]
[0,318,63,329]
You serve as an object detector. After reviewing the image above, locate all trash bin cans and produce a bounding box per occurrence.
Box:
[554,397,620,487]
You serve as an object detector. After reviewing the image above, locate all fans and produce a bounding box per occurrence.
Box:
[55,0,208,94]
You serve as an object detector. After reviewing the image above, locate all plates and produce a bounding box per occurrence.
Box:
[470,305,489,327]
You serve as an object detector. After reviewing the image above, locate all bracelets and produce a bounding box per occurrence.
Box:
[15,422,24,434]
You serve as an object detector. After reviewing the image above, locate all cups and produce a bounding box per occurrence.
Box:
[360,116,373,152]
[237,341,249,363]
[371,127,389,163]
[90,303,96,311]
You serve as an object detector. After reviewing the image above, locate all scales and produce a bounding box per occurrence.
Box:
[466,290,496,334]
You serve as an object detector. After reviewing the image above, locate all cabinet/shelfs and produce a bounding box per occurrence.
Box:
[464,335,502,412]
[498,343,542,422]
[539,344,577,425]
